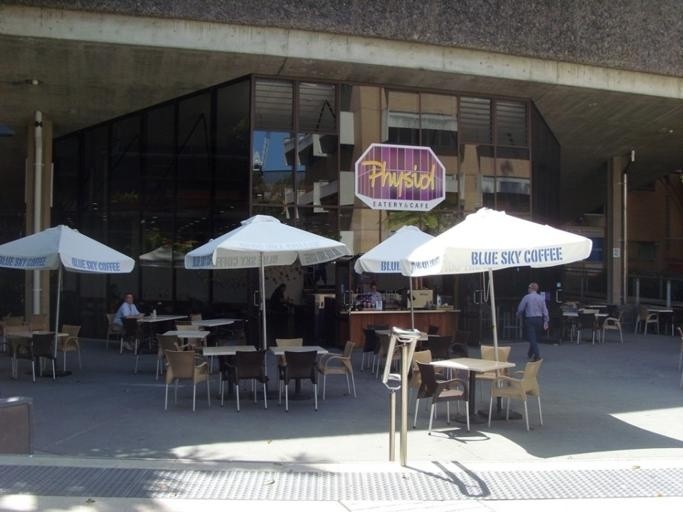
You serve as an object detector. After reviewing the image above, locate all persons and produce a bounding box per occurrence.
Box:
[268,284,289,316]
[368,283,382,304]
[516,282,550,361]
[112,292,145,352]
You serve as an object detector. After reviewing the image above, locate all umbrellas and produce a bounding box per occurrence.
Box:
[0,225,135,358]
[354,224,437,330]
[400,206,593,376]
[185,215,353,376]
[146,241,208,271]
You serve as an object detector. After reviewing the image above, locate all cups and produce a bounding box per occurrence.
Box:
[362,301,376,308]
